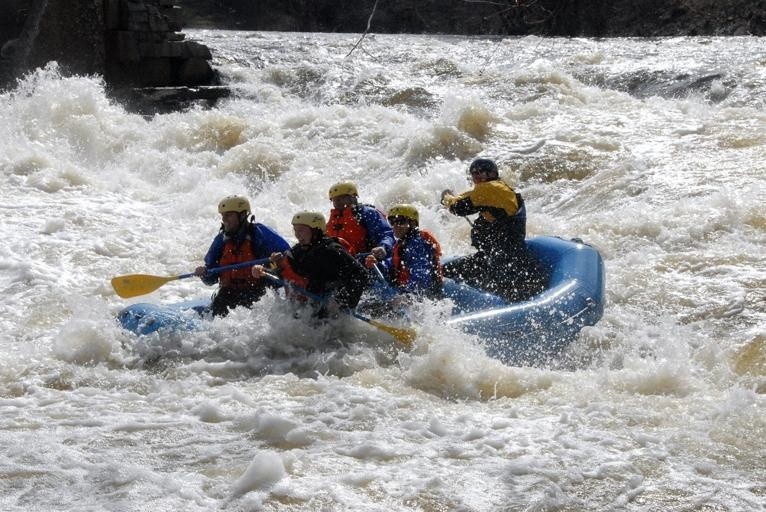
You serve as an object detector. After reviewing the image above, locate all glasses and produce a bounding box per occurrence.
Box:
[390,219,407,225]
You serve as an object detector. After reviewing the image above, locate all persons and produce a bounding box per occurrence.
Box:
[194,193,292,317]
[441,159,528,303]
[325,182,396,276]
[365,204,442,304]
[251,209,365,319]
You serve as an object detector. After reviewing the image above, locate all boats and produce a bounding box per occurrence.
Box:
[118,235,604,366]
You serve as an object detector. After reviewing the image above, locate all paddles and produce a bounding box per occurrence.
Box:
[111,255,282,298]
[261,271,417,350]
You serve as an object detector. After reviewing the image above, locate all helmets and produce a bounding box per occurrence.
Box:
[292,211,327,234]
[218,194,251,214]
[328,181,358,200]
[388,204,419,227]
[469,159,498,179]
